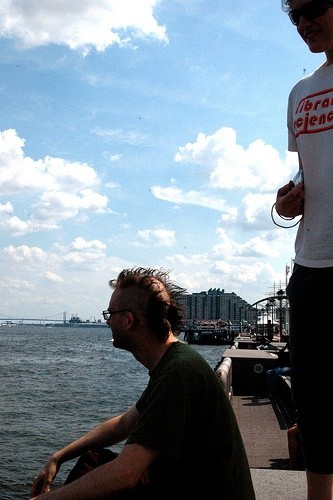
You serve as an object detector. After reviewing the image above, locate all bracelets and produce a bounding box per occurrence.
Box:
[270,203,304,228]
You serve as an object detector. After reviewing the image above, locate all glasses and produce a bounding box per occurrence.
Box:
[103,310,131,320]
[288,0,333,26]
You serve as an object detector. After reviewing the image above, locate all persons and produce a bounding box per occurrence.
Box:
[275,0,333,498]
[266,320,274,342]
[31,271,255,500]
